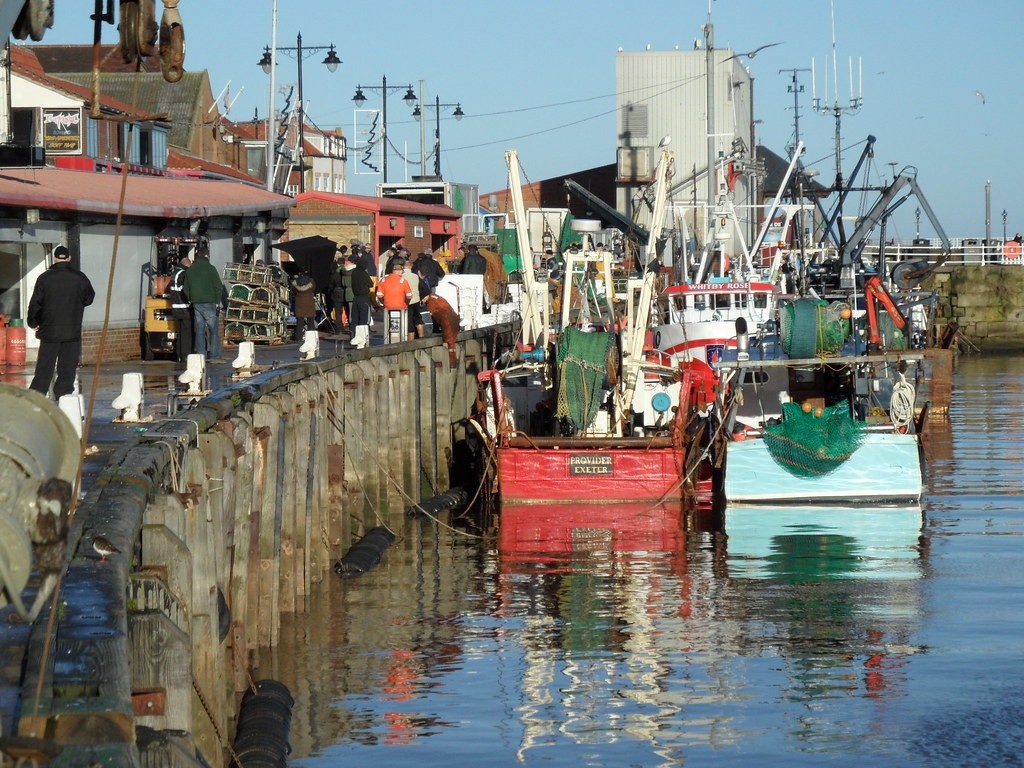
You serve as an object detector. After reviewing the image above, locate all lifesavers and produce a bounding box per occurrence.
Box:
[1004,240,1021,259]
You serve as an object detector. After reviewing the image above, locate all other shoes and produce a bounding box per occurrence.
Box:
[210,358,227,363]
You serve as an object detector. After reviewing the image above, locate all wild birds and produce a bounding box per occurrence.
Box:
[973,88,987,105]
[718,41,786,64]
[658,133,671,151]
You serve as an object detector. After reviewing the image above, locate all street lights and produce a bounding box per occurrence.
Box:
[351,74,420,182]
[913,206,922,245]
[255,31,346,195]
[1000,209,1008,244]
[411,95,466,176]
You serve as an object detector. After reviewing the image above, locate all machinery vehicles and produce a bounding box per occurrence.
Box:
[136,229,212,361]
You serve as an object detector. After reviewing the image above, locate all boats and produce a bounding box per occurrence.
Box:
[456,0,952,514]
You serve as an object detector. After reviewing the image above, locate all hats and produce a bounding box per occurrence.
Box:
[393,265,403,271]
[546,250,553,254]
[54,246,69,260]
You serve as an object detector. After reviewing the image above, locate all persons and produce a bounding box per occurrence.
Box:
[292,264,316,340]
[255,260,281,280]
[319,239,377,339]
[376,244,486,342]
[563,242,623,265]
[782,253,802,294]
[28,246,95,403]
[165,247,226,364]
[546,251,560,313]
[1013,233,1024,246]
